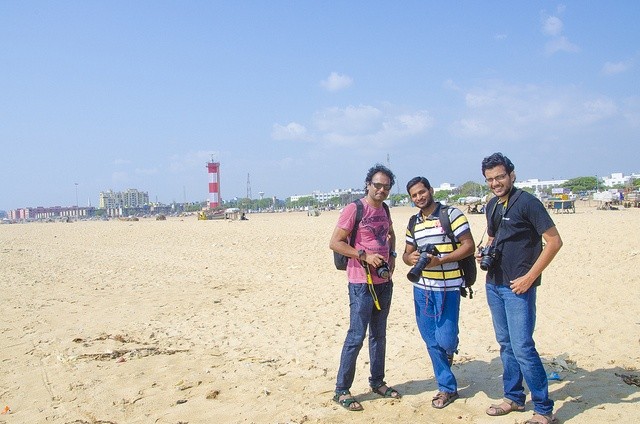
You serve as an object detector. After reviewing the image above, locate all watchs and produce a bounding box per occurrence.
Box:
[358,250,365,259]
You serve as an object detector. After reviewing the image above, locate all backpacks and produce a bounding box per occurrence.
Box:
[333,198,390,270]
[409,206,476,286]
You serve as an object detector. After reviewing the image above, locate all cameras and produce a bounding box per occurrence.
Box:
[479,246,500,271]
[407,244,441,283]
[376,259,390,278]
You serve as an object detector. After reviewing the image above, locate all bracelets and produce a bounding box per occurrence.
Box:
[390,252,396,257]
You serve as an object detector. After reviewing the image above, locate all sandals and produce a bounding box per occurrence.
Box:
[372,382,400,398]
[486,398,525,416]
[432,391,459,409]
[333,390,362,411]
[524,411,558,424]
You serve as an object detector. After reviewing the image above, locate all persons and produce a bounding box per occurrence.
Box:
[403,177,476,409]
[474,153,563,424]
[241,213,246,219]
[329,165,400,411]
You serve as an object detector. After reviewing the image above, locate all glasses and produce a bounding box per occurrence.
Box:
[485,172,507,183]
[369,180,391,191]
